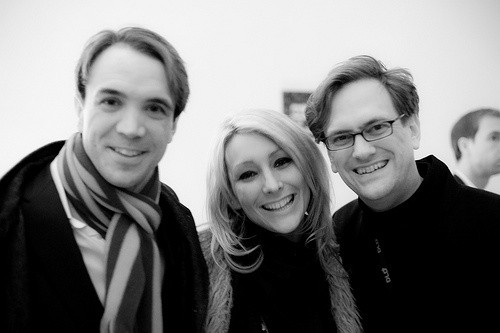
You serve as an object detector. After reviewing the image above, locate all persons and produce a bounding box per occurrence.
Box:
[304,55,500,333]
[0,25,211,333]
[194,109,363,333]
[448,107,500,195]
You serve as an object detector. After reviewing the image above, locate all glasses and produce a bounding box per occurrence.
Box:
[319,113,406,151]
